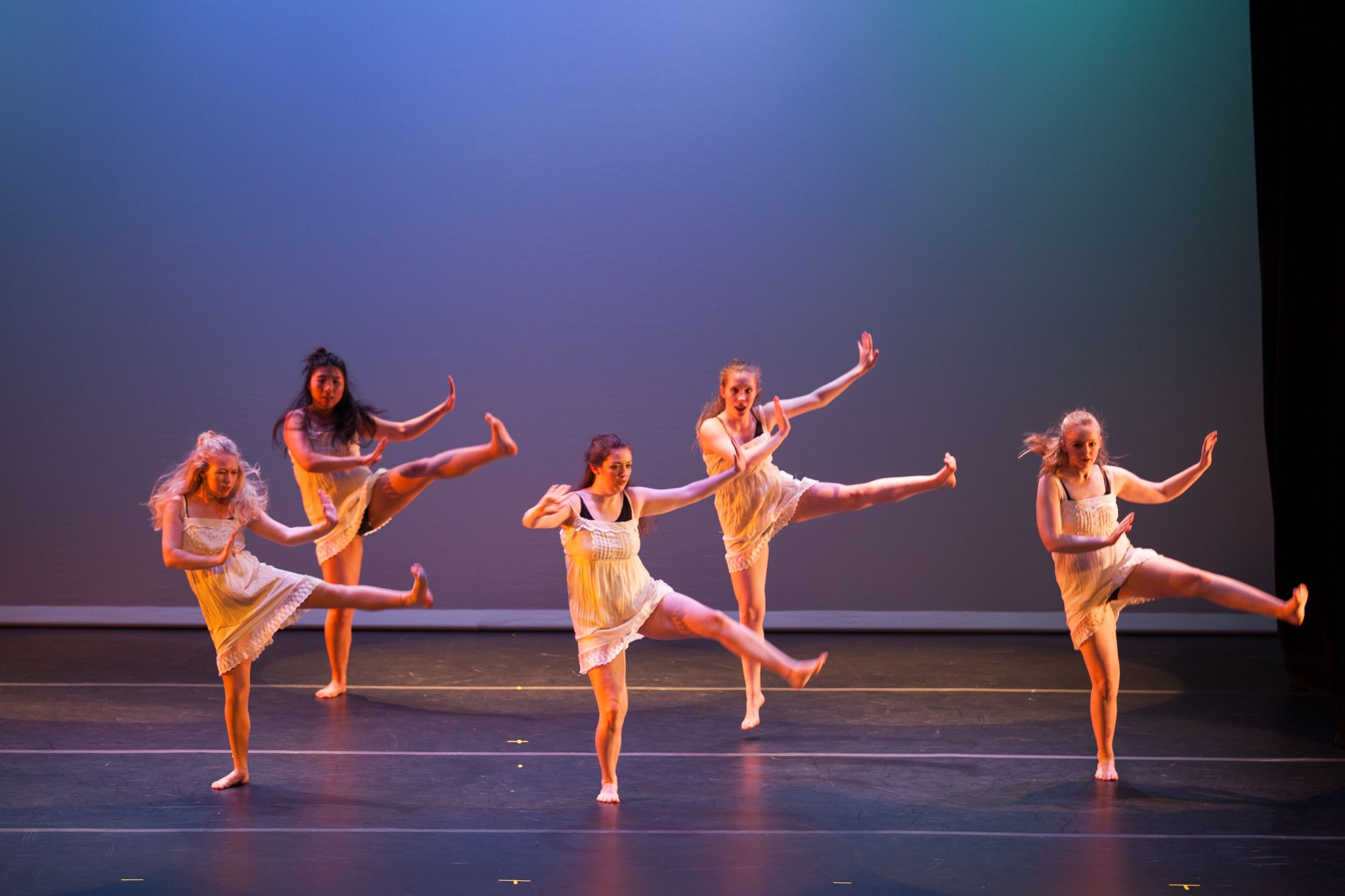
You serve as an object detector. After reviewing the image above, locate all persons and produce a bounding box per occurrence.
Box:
[271,347,519,699]
[1018,409,1309,782]
[696,331,957,731]
[521,432,829,804]
[140,429,434,790]
